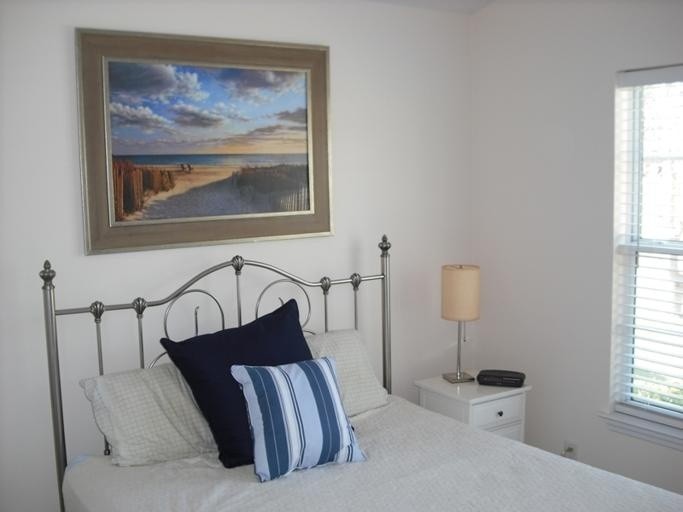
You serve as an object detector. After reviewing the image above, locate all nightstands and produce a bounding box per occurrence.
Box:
[413,372,534,445]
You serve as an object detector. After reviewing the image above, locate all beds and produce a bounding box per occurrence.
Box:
[38,235,679,511]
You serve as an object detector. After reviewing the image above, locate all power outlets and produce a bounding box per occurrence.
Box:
[565,444,577,460]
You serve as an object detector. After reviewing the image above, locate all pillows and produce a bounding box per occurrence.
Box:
[230,354,367,482]
[301,326,390,419]
[161,297,314,469]
[78,360,218,469]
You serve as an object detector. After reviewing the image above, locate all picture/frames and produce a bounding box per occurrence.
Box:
[74,27,334,255]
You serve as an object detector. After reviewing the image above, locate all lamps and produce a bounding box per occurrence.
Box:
[440,262,481,385]
